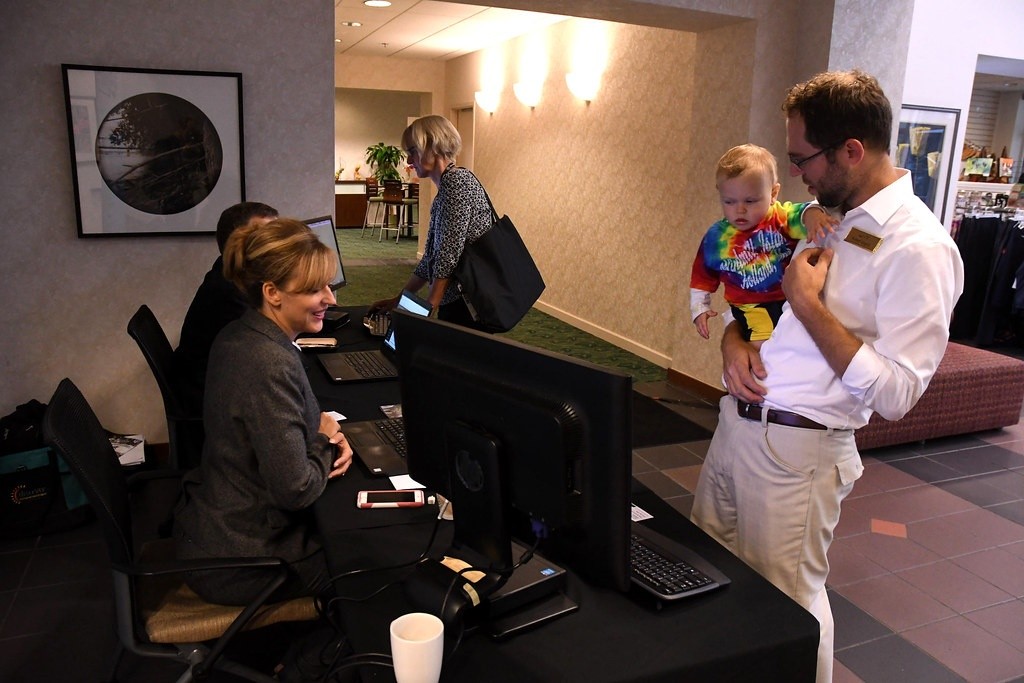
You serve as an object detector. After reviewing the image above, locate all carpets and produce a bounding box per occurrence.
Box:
[335,262,667,385]
[337,227,418,259]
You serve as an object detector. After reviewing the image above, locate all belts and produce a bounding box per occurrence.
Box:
[737,398,846,431]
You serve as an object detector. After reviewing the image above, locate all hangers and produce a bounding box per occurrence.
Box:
[964,204,1024,230]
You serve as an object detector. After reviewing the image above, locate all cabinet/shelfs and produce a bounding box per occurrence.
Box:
[336,180,368,229]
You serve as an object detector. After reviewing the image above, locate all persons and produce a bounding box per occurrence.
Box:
[367,115,496,333]
[690,144,840,391]
[169,217,353,683]
[171,202,279,477]
[690,69,963,683]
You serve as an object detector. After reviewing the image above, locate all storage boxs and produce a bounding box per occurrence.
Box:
[0,446,98,540]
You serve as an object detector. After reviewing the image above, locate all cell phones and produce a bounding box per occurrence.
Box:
[356,490,425,508]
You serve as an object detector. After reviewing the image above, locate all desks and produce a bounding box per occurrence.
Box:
[369,197,419,239]
[297,304,821,683]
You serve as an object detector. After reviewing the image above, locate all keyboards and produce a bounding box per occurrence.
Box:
[630,519,732,600]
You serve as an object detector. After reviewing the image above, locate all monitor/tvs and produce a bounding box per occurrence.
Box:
[387,308,634,639]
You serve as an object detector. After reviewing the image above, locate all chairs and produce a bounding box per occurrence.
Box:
[126,305,201,481]
[42,378,327,683]
[361,178,419,244]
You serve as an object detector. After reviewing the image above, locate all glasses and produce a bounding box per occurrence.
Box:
[405,146,415,157]
[790,134,864,172]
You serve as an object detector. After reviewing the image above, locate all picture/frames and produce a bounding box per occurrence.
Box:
[61,62,246,239]
[894,103,961,226]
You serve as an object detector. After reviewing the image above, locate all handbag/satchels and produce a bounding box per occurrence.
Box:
[452,214,547,333]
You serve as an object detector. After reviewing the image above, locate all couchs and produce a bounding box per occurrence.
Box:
[855,341,1024,457]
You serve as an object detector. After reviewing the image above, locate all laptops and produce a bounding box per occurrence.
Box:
[318,287,435,382]
[341,417,410,476]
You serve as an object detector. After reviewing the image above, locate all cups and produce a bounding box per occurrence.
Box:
[390,612,445,683]
